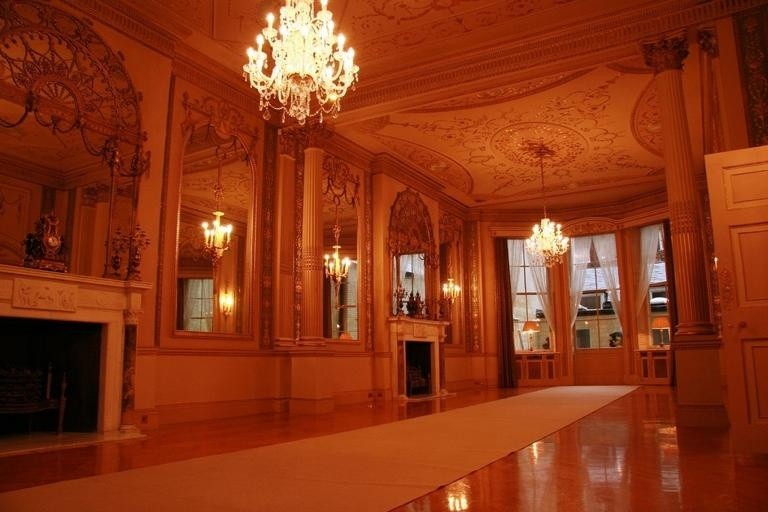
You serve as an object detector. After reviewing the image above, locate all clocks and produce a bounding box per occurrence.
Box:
[19,220,39,268]
[34,209,68,273]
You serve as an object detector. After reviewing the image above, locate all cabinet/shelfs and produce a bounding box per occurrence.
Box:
[514,351,561,387]
[634,349,674,386]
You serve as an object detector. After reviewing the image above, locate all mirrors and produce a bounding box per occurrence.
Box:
[389,186,437,318]
[317,172,362,346]
[171,120,259,343]
[0,0,146,278]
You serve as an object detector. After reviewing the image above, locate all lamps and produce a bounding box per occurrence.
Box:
[323,204,351,287]
[201,145,233,262]
[523,147,570,268]
[220,279,234,322]
[522,320,541,352]
[650,316,670,345]
[442,278,460,300]
[243,0,360,127]
